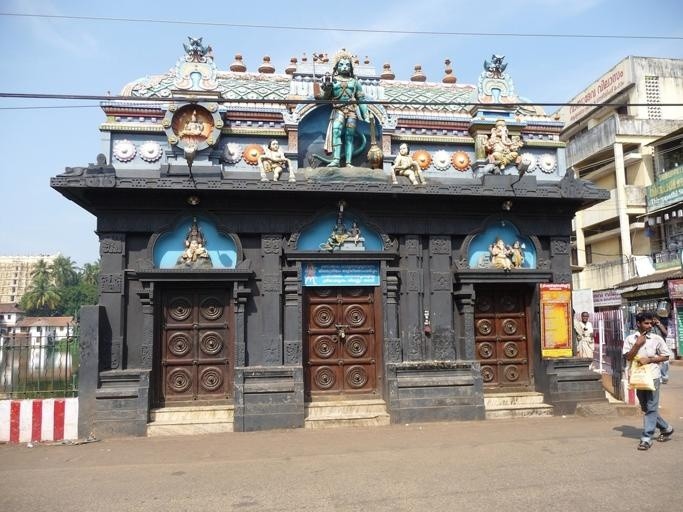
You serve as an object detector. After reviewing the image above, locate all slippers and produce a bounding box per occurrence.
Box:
[637,440,653,450]
[656,428,674,442]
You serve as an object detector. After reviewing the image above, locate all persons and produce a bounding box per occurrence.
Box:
[489,240,511,270]
[263,138,286,181]
[622,311,675,451]
[394,142,419,185]
[576,311,596,370]
[313,50,376,167]
[183,224,209,259]
[331,218,347,236]
[346,222,361,244]
[573,309,582,355]
[650,312,669,385]
[507,240,524,268]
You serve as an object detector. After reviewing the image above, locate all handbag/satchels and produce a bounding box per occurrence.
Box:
[628,356,657,392]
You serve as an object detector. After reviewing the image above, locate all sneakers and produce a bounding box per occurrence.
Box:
[662,379,669,385]
